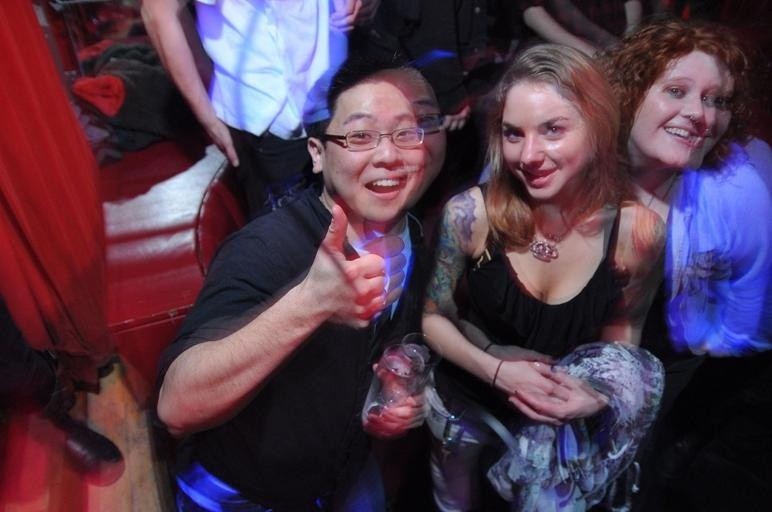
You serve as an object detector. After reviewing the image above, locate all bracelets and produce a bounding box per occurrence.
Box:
[492,360,505,386]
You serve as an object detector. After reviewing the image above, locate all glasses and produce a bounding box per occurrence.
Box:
[421,115,443,133]
[319,128,424,151]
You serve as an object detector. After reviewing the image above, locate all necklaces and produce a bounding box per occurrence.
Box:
[529,200,595,260]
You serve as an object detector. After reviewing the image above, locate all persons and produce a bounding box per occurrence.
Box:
[423,43,667,511]
[139,0,362,219]
[611,24,771,512]
[367,2,672,219]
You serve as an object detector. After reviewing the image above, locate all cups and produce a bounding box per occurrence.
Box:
[396,329,442,391]
[361,344,423,431]
